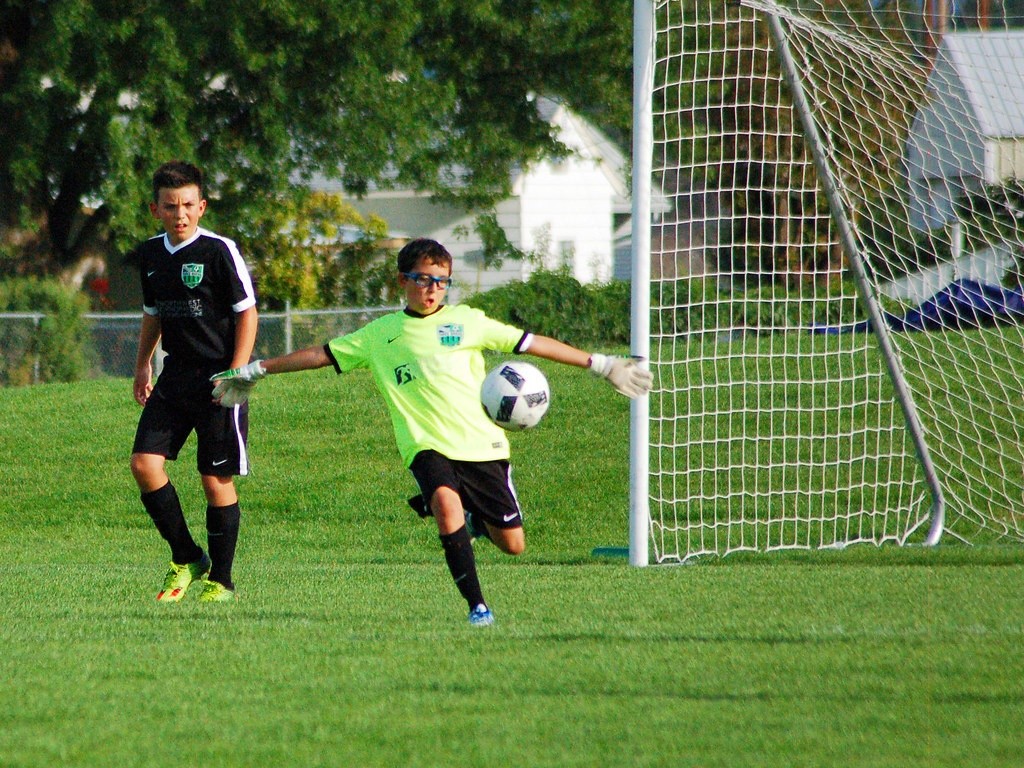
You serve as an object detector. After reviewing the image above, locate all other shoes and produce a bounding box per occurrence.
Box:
[469,606,493,627]
[463,509,472,538]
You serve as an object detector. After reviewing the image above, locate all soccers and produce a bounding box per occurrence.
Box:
[481,359,552,432]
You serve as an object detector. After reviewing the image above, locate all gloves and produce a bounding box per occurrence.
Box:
[208,360,266,408]
[590,353,653,400]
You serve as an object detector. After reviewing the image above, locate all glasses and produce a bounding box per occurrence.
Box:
[404,272,452,289]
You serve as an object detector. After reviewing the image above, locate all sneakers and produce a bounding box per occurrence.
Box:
[156,548,210,602]
[201,580,236,601]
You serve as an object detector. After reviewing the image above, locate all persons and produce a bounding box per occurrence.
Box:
[210,238,656,629]
[127,158,258,606]
